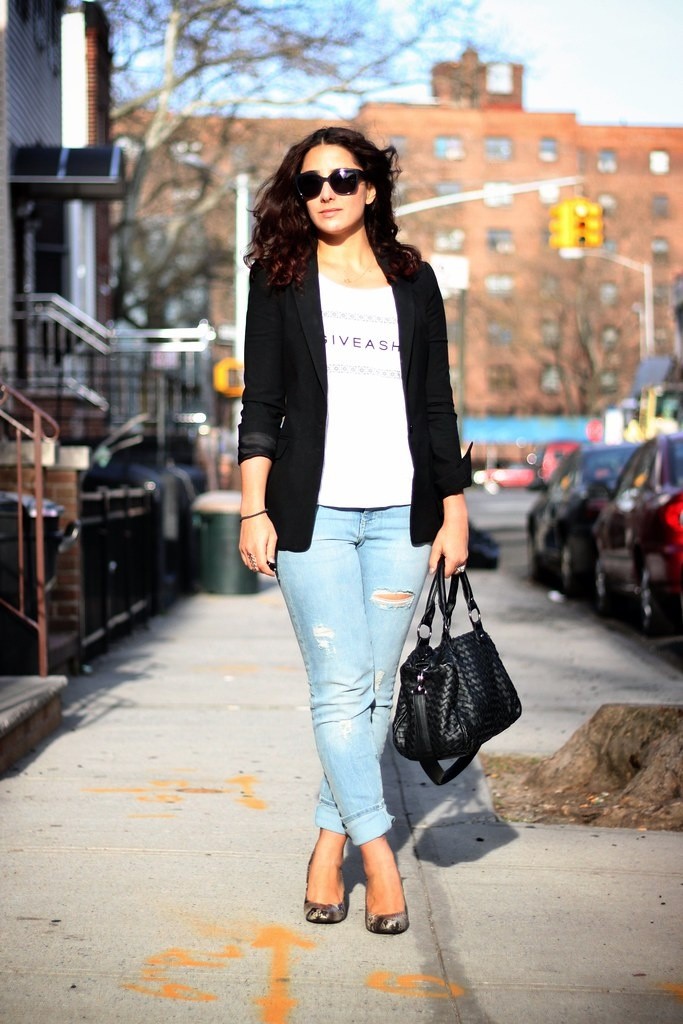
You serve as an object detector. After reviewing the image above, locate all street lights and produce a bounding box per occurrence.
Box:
[560,246,653,360]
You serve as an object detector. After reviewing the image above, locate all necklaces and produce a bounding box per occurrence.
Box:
[344,259,375,284]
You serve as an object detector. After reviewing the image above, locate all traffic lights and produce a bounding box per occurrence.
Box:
[566,197,592,247]
[587,204,606,247]
[550,203,568,250]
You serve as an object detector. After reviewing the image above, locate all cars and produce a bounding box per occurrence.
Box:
[590,431,683,639]
[529,440,649,606]
[469,438,583,495]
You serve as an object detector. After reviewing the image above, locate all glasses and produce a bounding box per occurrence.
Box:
[293,169,368,206]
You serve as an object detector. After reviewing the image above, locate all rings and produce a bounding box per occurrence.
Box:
[455,565,465,574]
[249,555,258,571]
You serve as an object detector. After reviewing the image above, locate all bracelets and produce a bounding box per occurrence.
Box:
[240,509,268,521]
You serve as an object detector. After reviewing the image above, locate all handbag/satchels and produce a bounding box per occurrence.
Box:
[393,555,523,786]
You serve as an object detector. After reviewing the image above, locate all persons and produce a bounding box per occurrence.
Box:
[237,126,472,935]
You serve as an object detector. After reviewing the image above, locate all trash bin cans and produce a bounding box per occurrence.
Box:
[189,488,259,594]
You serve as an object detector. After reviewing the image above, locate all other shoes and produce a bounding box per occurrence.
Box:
[365,868,409,936]
[305,860,349,923]
